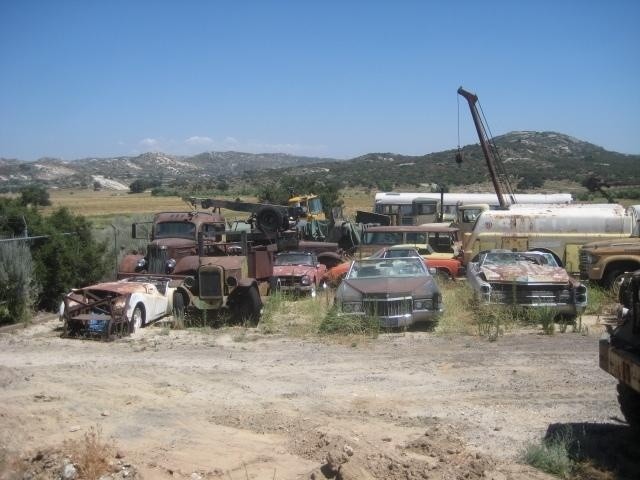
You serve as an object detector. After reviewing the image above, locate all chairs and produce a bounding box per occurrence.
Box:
[357,265,381,277]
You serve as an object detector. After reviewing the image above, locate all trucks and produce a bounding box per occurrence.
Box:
[373,189,574,243]
[449,88,640,280]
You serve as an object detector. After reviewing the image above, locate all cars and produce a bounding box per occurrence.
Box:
[326,242,461,291]
[467,247,587,321]
[322,242,447,333]
